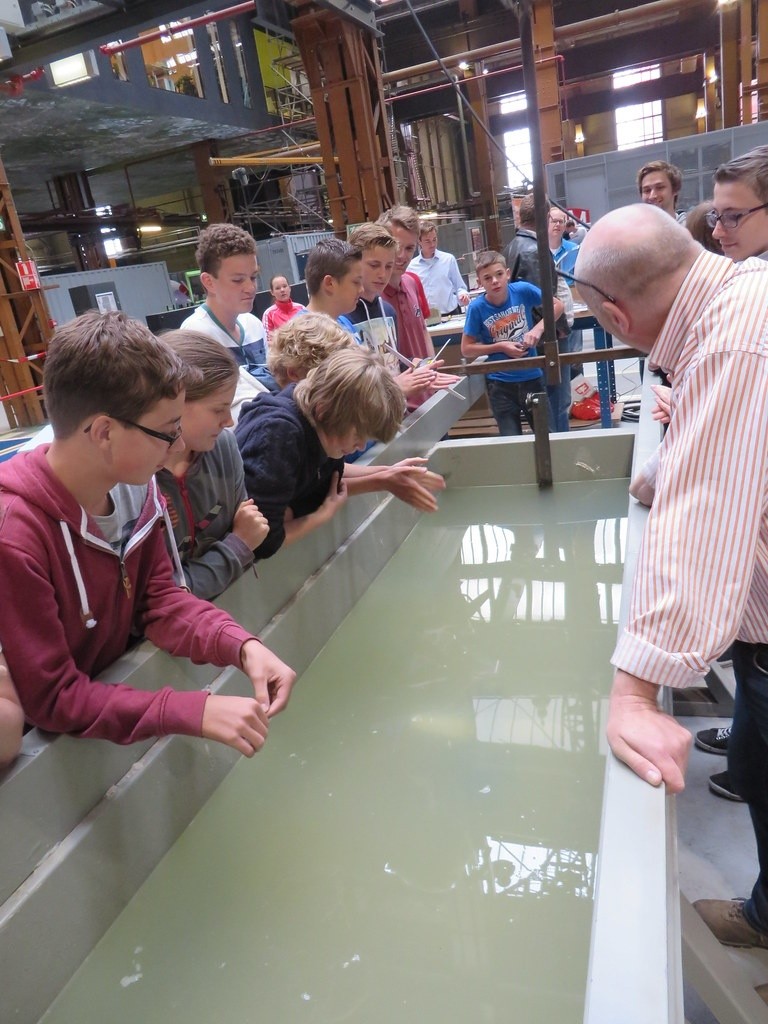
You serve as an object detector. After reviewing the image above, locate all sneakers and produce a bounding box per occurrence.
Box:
[709,771,745,803]
[695,726,732,754]
[691,897,768,948]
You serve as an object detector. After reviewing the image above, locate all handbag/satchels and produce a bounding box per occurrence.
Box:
[567,372,615,421]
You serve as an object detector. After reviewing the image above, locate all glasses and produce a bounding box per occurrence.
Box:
[550,219,567,224]
[555,244,616,307]
[81,415,183,450]
[704,203,768,228]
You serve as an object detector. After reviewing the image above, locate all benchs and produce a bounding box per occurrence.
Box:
[447,403,623,438]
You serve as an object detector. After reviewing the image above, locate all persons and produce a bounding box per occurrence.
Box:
[181,204,470,513]
[233,349,407,558]
[0,310,298,757]
[460,194,586,435]
[157,330,270,603]
[572,202,768,1009]
[636,144,768,801]
[0,643,24,767]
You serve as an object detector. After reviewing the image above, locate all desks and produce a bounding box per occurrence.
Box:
[426,296,617,428]
